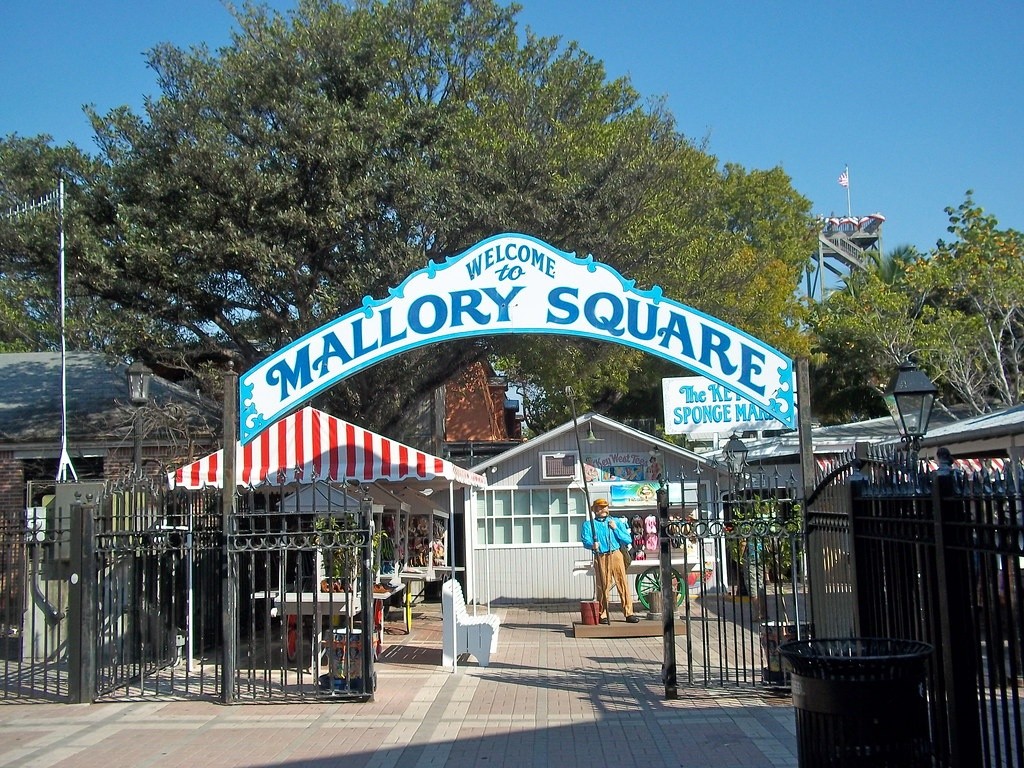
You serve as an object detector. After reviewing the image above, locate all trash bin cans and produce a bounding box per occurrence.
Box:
[782,635,937,768]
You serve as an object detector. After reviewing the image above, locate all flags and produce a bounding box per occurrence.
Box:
[817,214,885,228]
[838,170,848,186]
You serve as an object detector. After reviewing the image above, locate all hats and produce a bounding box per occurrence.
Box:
[590,499,609,511]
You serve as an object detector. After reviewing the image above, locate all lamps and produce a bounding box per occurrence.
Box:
[490,466,498,474]
[653,445,661,456]
[482,468,488,476]
[581,421,605,444]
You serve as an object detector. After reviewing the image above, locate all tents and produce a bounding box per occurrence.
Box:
[168,407,490,674]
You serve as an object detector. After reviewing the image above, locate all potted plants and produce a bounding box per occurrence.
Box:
[727,494,811,672]
[313,513,398,677]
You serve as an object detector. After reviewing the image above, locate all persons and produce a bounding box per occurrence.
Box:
[738,511,770,621]
[581,498,639,623]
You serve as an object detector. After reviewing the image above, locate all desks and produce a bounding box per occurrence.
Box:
[251,566,465,672]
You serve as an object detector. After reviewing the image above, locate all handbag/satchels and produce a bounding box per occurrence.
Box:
[620,548,631,570]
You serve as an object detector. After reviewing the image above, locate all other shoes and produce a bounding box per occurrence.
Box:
[626,615,638,623]
[599,617,608,623]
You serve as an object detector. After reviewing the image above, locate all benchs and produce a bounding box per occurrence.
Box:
[440,579,500,665]
